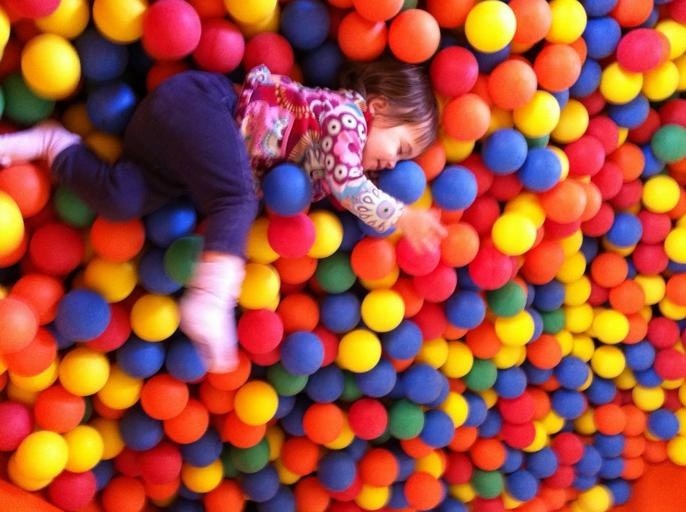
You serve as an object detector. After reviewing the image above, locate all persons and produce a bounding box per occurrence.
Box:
[1,59,447,373]
